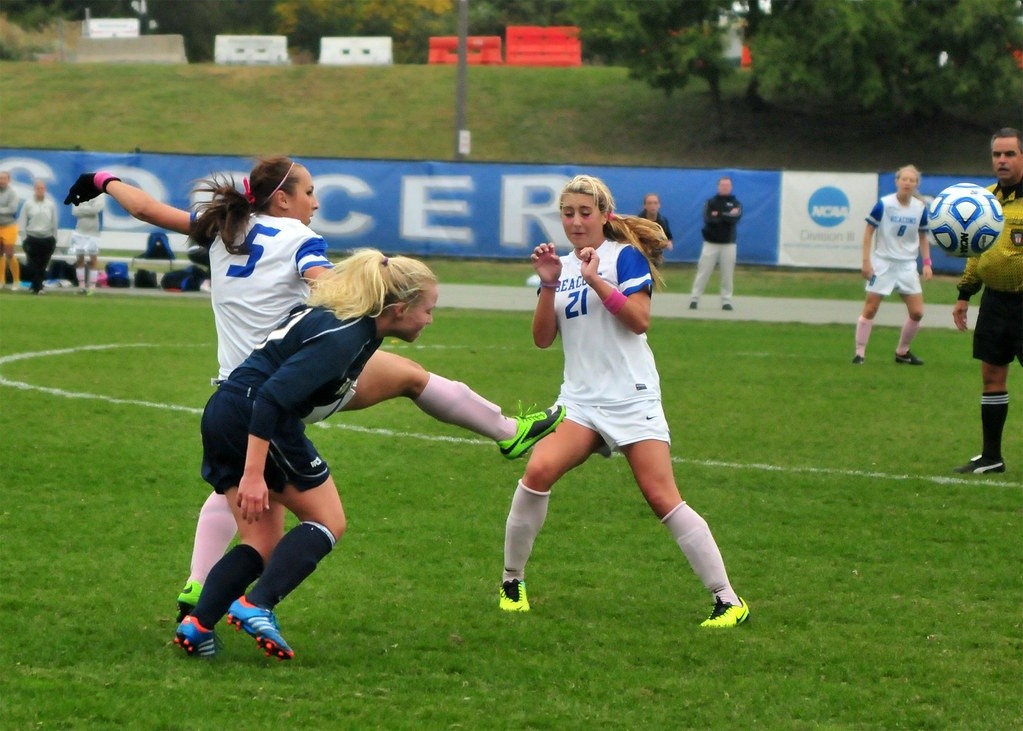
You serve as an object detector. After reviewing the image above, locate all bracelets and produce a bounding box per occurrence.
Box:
[540,281,561,287]
[93,170,116,191]
[602,288,627,314]
[922,258,932,266]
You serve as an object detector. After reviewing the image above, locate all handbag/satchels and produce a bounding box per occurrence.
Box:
[160,264,206,290]
[135,268,158,288]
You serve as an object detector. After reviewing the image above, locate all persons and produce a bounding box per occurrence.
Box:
[18,182,57,295]
[72,193,102,295]
[0,171,19,291]
[638,193,673,267]
[689,177,742,310]
[500,174,749,628]
[173,247,439,661]
[851,164,933,365]
[64,154,565,622]
[952,128,1023,474]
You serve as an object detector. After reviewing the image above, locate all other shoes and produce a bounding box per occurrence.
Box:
[689,301,698,309]
[721,303,732,310]
[73,288,86,295]
[87,287,96,296]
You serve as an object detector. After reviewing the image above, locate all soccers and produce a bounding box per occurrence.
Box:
[926,182,1004,257]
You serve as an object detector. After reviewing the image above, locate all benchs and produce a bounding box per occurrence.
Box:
[11,253,206,272]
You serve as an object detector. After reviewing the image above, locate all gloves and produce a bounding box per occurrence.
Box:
[64,172,120,206]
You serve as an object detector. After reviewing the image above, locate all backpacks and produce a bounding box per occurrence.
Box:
[106,261,130,287]
[145,232,175,260]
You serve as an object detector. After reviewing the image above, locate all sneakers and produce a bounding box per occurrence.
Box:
[497,404,567,459]
[895,350,924,366]
[954,455,1005,474]
[499,577,529,612]
[175,582,203,623]
[852,356,865,364]
[174,616,217,656]
[227,596,296,661]
[699,596,752,627]
